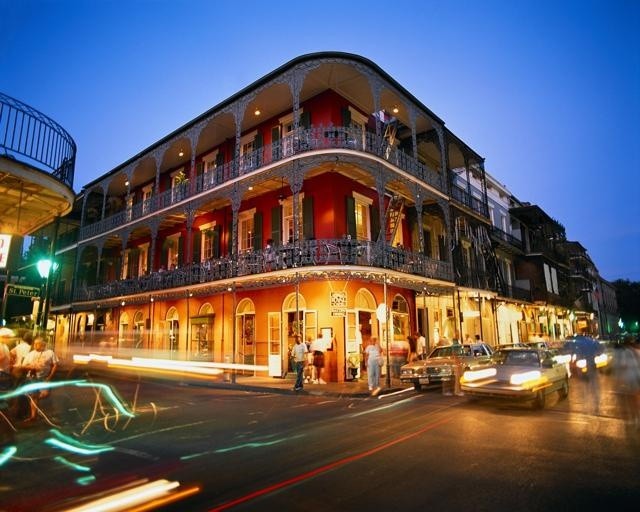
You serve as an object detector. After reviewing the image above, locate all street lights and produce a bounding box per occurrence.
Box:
[31,254,64,338]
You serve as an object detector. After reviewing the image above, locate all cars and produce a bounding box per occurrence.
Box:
[483,329,640,377]
[457,343,572,409]
[398,340,494,396]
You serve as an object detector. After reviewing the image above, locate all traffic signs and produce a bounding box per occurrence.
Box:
[5,284,40,298]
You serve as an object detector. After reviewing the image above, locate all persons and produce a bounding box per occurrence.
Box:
[475,334,482,344]
[414,332,426,360]
[291,333,308,393]
[309,332,336,384]
[0,327,60,423]
[359,323,363,353]
[406,335,416,363]
[266,238,287,269]
[439,340,445,346]
[364,336,383,391]
[465,334,474,343]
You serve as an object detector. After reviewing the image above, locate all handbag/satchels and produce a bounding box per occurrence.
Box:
[376,356,384,367]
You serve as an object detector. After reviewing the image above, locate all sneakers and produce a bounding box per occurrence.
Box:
[295,377,327,391]
